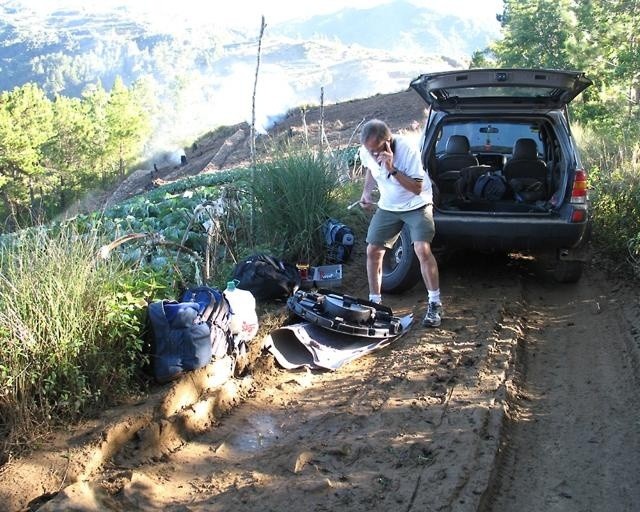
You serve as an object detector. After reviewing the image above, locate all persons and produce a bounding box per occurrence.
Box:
[358,118,444,328]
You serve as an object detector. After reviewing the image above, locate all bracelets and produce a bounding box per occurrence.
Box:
[387,168,398,178]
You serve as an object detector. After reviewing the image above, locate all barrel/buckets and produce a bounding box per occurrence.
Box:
[223,279,259,339]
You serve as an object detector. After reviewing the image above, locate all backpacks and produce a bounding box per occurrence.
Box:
[321,217,355,266]
[146,297,213,385]
[179,284,235,363]
[232,253,302,305]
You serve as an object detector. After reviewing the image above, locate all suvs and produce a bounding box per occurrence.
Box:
[400,62,593,285]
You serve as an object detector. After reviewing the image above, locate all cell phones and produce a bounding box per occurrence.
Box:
[386,138,393,153]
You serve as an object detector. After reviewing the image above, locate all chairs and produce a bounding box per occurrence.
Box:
[503,137,546,180]
[438,135,479,170]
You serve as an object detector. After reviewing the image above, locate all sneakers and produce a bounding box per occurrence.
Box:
[421,301,445,328]
